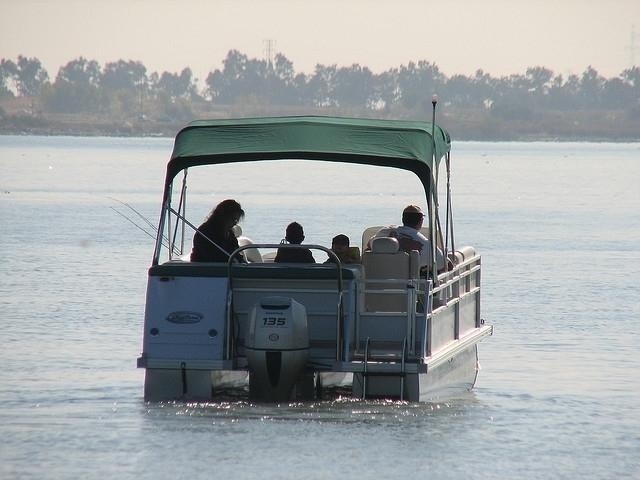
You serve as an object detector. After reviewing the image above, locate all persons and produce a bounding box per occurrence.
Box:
[273,222,316,264]
[323,234,355,263]
[365,203,455,274]
[190,198,246,263]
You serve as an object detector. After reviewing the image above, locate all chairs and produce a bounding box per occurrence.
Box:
[419,225,443,251]
[230,225,262,265]
[358,235,420,315]
[362,226,385,248]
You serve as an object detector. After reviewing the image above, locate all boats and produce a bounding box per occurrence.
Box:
[133,99,494,405]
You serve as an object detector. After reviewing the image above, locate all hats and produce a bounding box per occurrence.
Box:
[402,205,425,214]
